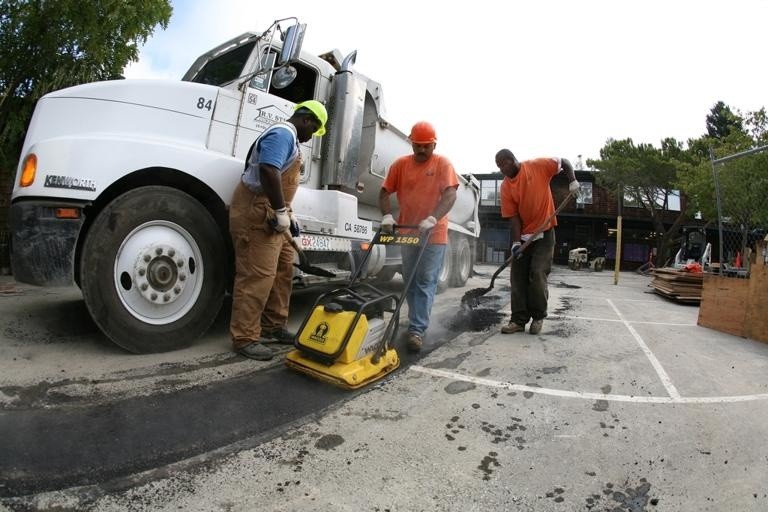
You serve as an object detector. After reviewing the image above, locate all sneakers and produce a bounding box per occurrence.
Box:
[232,340,274,361]
[529,316,543,334]
[406,329,423,352]
[258,325,297,344]
[500,320,526,333]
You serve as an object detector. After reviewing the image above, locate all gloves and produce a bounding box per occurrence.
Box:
[289,210,300,237]
[416,215,438,236]
[272,205,292,234]
[510,241,524,262]
[568,179,582,200]
[381,214,400,237]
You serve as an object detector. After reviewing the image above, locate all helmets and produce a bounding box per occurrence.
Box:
[294,99,329,138]
[407,121,438,146]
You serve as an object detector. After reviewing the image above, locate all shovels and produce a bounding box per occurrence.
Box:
[266,216,336,277]
[461,186,581,300]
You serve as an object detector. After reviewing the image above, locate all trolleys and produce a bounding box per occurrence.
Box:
[284,224,432,390]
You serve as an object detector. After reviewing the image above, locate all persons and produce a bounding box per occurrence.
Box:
[494,148,581,334]
[378,120,460,354]
[227,98,329,360]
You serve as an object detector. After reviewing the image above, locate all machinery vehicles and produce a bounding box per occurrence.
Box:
[10,16,481,355]
